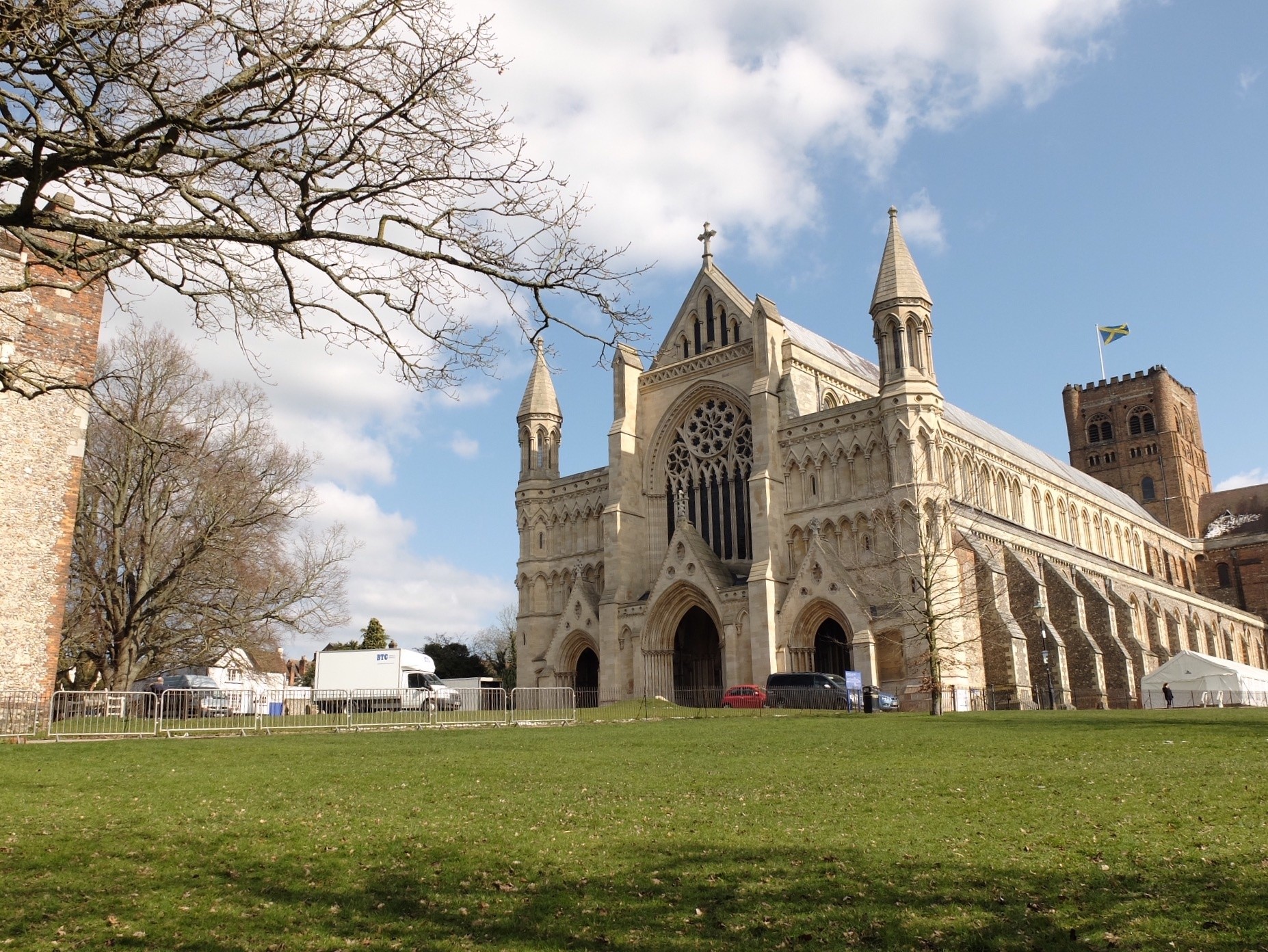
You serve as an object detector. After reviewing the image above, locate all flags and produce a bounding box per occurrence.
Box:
[1098,323,1130,345]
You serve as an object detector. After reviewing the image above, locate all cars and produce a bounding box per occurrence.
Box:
[721,684,767,709]
[855,683,900,711]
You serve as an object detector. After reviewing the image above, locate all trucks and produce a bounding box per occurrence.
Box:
[313,648,462,712]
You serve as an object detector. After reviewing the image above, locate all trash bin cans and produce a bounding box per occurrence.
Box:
[863,685,880,713]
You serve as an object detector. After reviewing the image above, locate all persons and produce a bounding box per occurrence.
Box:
[1162,683,1174,709]
[150,677,167,718]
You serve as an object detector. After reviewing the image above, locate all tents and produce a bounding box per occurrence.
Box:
[1140,649,1267,710]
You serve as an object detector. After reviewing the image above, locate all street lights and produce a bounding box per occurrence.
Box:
[1034,597,1055,711]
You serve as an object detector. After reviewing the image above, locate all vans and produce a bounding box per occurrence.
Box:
[766,671,866,710]
[163,674,232,715]
